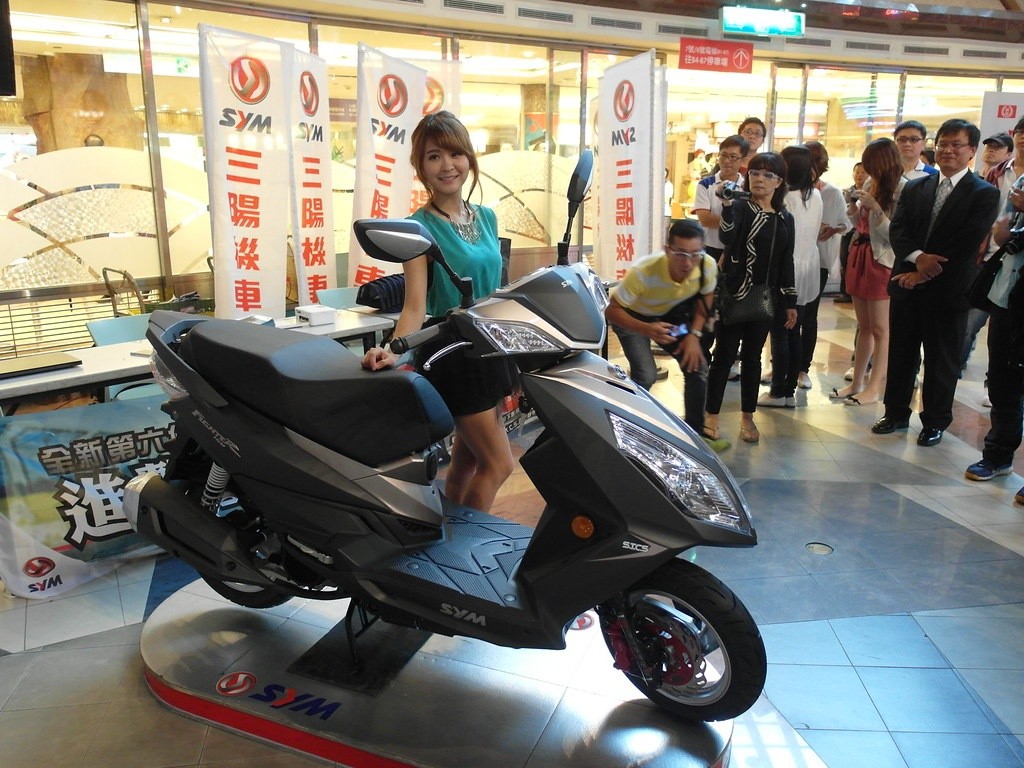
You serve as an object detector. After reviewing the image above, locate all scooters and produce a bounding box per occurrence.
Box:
[121,149,768,723]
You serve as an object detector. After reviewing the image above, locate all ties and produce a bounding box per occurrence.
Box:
[927,177,950,239]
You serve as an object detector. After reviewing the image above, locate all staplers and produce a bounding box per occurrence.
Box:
[180,291,200,300]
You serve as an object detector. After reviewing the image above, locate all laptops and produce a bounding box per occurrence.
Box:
[0,352,83,379]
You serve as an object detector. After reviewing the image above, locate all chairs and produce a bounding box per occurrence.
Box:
[84,313,165,401]
[314,286,360,311]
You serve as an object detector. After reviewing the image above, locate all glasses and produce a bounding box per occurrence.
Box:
[669,249,704,262]
[740,130,763,137]
[718,153,743,162]
[934,142,970,151]
[894,135,923,144]
[748,169,781,181]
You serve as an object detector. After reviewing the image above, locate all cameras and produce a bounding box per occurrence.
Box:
[850,190,862,202]
[1004,228,1024,255]
[722,182,752,201]
[667,323,689,337]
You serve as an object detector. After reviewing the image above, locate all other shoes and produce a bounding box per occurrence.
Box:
[702,436,730,451]
[845,367,870,379]
[760,370,772,382]
[785,396,796,407]
[833,296,853,303]
[756,393,785,407]
[727,368,741,382]
[797,375,812,388]
[981,386,992,407]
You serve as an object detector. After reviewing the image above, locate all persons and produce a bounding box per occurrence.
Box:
[761,143,850,387]
[826,119,1024,503]
[835,163,871,302]
[664,168,675,246]
[605,219,731,452]
[738,117,766,357]
[689,134,751,383]
[757,147,822,406]
[703,152,798,444]
[358,106,516,512]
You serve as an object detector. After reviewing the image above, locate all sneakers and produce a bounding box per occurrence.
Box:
[1014,487,1024,503]
[965,459,1014,480]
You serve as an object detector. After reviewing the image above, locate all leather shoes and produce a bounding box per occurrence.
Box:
[872,416,910,433]
[917,427,943,446]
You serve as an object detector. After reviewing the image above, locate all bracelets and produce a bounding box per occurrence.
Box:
[872,211,884,221]
[690,328,703,337]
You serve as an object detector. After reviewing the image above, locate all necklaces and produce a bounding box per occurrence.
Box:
[428,197,482,247]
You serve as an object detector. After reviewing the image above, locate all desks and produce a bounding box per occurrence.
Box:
[0,308,393,401]
[344,306,433,326]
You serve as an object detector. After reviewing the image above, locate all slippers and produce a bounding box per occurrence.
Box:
[844,396,878,406]
[830,388,859,398]
[740,426,758,442]
[702,425,719,440]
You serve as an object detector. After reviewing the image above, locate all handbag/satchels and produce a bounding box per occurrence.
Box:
[966,259,1004,313]
[720,285,773,328]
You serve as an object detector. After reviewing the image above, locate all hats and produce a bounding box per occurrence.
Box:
[983,133,1014,152]
